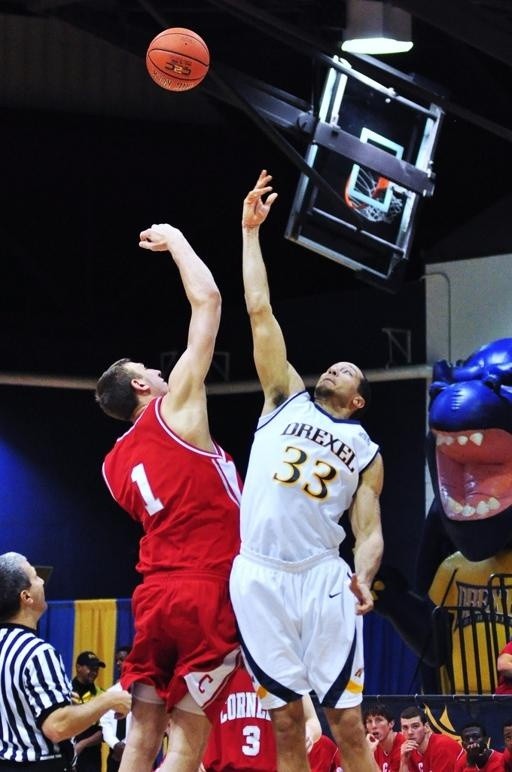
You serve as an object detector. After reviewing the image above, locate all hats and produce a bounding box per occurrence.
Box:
[77,651,106,668]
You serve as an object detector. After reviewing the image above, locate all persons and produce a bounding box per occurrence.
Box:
[228,167,386,771]
[0,552,133,772]
[69,640,510,772]
[94,222,244,772]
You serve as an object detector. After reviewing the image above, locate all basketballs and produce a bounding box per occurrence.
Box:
[146,28,210,93]
